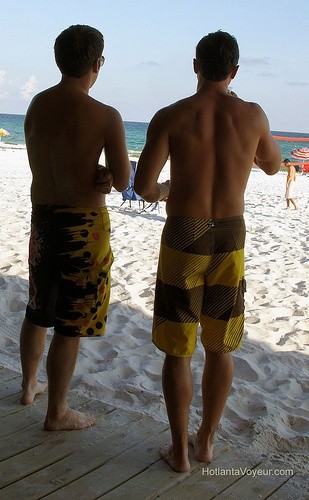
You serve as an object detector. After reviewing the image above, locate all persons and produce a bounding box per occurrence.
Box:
[282,159,298,210]
[17,23,129,429]
[133,26,286,475]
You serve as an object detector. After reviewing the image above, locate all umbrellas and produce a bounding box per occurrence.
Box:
[289,146,309,176]
[0,127,15,138]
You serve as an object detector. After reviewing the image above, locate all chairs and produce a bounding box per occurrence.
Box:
[122,158,163,219]
